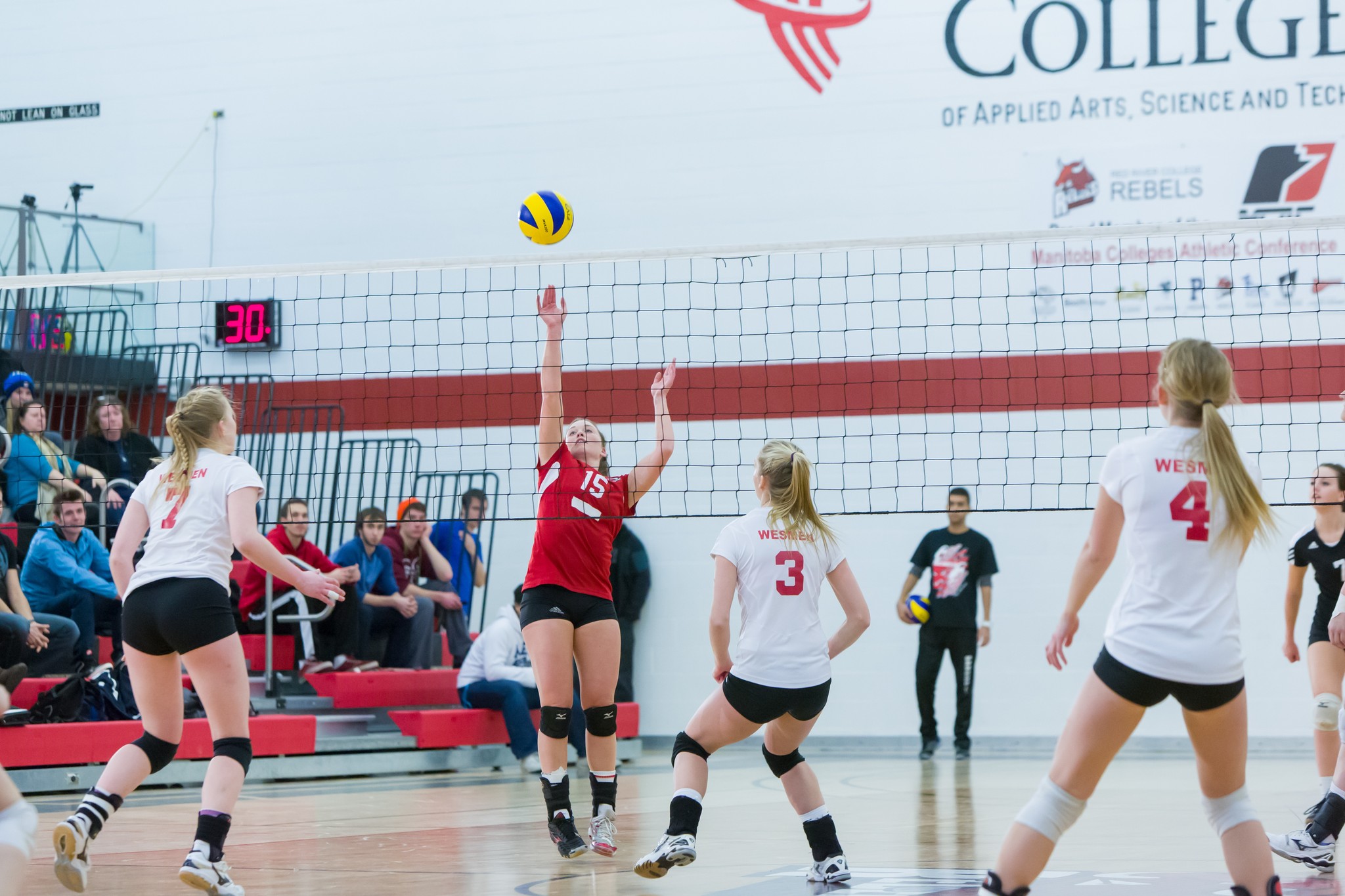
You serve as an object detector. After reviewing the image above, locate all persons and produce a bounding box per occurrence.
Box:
[455,584,579,774]
[0,370,163,727]
[430,489,488,626]
[1269,464,1345,873]
[381,498,472,669]
[327,507,418,668]
[0,765,40,896]
[974,336,1286,896]
[520,285,676,859]
[53,386,347,896]
[632,439,871,885]
[609,523,650,703]
[237,499,379,674]
[896,487,998,759]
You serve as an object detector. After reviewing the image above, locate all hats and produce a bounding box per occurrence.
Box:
[394,498,421,528]
[3,371,36,397]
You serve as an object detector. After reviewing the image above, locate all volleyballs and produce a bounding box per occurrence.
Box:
[905,595,931,623]
[518,190,574,245]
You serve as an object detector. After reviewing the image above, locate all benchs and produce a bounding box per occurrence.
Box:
[0,523,641,794]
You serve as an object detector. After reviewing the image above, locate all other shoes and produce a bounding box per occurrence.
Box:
[576,758,621,770]
[521,753,542,775]
[955,746,970,760]
[336,656,379,672]
[297,659,332,686]
[918,737,940,760]
[1304,798,1327,823]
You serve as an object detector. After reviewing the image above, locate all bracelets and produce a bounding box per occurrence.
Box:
[980,619,991,628]
[28,619,36,622]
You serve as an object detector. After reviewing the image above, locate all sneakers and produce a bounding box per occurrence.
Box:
[52,816,90,893]
[547,813,587,861]
[633,831,697,879]
[588,804,617,857]
[179,849,246,896]
[805,855,852,885]
[1272,824,1337,873]
